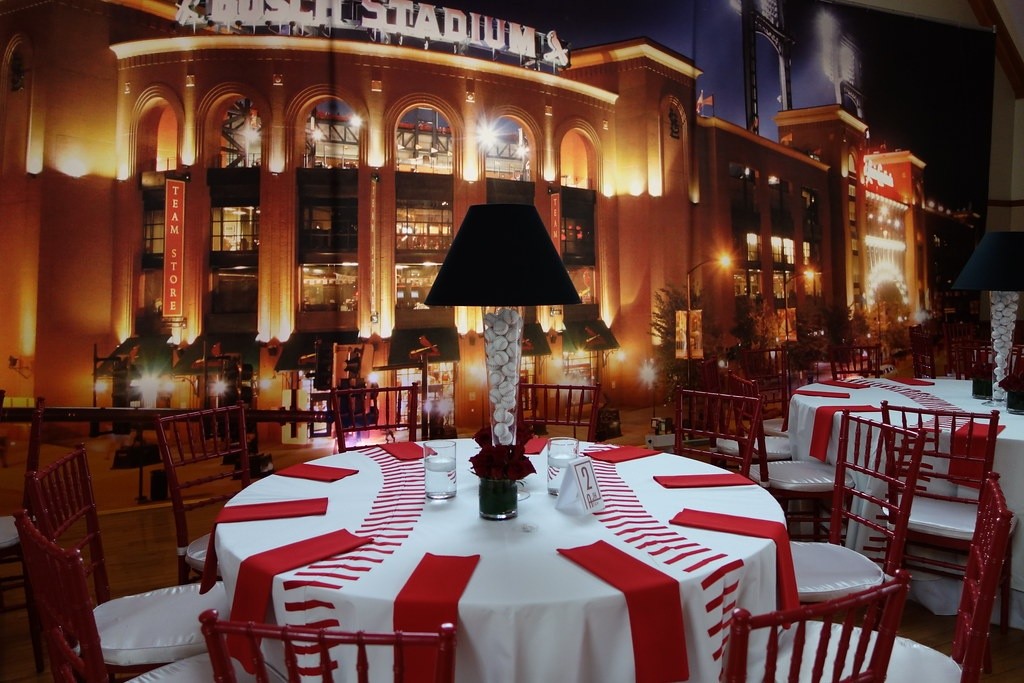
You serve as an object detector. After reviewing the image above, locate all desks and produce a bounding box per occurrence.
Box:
[214,437,786,682]
[789,377,1024,633]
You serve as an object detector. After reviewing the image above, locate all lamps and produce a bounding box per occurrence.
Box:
[424,204,581,500]
[952,232,1024,408]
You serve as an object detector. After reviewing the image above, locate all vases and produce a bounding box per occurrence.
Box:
[1006,389,1024,416]
[477,478,518,520]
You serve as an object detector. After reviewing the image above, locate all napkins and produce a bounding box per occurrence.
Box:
[653,473,756,488]
[809,405,883,462]
[584,445,663,463]
[816,380,870,389]
[669,509,802,630]
[378,442,437,462]
[226,529,373,675]
[199,498,328,594]
[524,436,548,454]
[781,390,850,433]
[393,553,480,683]
[887,377,935,386]
[556,540,690,682]
[947,421,1005,489]
[274,463,359,482]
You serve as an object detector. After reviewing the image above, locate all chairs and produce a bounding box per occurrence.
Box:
[762,471,1011,683]
[718,569,911,683]
[787,409,928,629]
[672,384,765,478]
[827,345,882,379]
[516,382,602,442]
[745,344,791,436]
[726,369,856,545]
[909,319,1024,380]
[701,354,792,469]
[0,381,458,683]
[876,400,1020,637]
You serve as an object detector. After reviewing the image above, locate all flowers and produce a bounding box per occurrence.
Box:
[998,373,1024,393]
[469,429,538,486]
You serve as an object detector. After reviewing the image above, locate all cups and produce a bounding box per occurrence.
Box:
[424,441,456,499]
[547,437,579,495]
